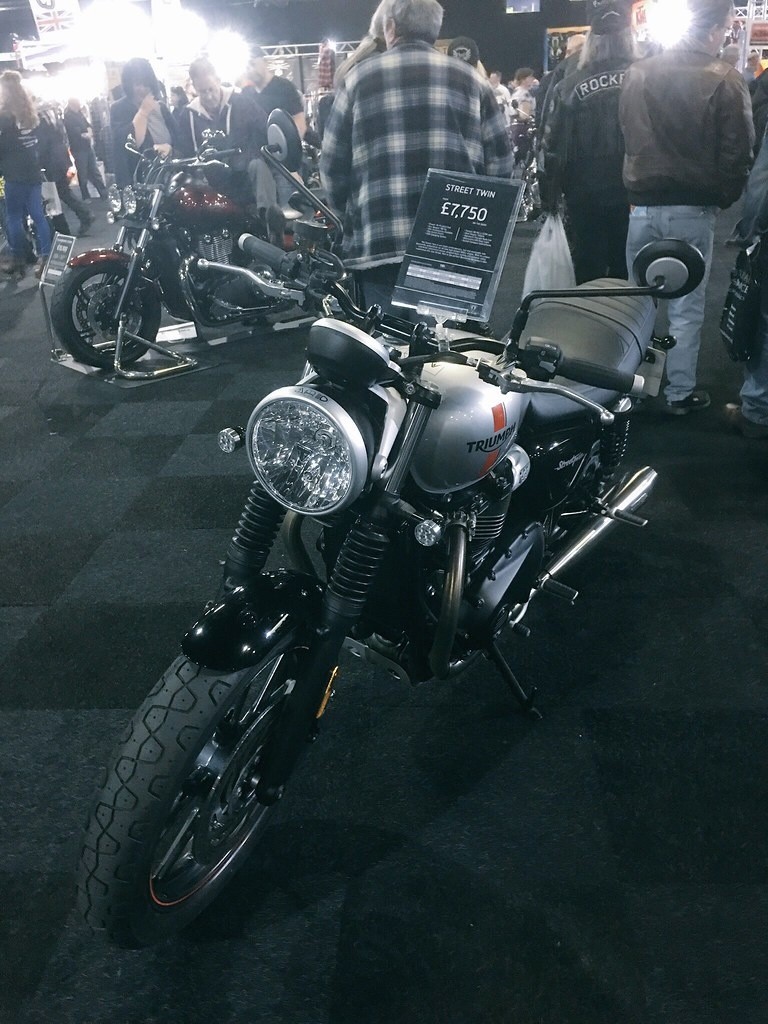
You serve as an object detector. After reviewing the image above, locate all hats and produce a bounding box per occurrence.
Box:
[591,6,630,35]
[446,36,479,64]
[746,53,759,59]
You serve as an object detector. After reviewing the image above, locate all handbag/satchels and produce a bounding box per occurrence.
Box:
[518,213,576,303]
[42,173,62,216]
[717,242,764,361]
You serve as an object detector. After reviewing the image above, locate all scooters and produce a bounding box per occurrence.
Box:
[507,98,547,221]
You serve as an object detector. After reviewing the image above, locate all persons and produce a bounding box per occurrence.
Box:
[535,0,768,429]
[0,64,114,278]
[317,0,535,320]
[105,47,310,250]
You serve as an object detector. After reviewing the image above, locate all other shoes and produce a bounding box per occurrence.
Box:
[77,213,96,235]
[723,403,767,439]
[265,206,285,248]
[669,390,710,415]
[81,198,92,205]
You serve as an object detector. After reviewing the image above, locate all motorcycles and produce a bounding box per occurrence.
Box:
[44,134,346,384]
[78,235,714,966]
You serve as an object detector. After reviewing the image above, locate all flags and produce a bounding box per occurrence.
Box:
[29,0,82,41]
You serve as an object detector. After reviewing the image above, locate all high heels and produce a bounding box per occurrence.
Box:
[34,261,45,278]
[2,260,26,279]
